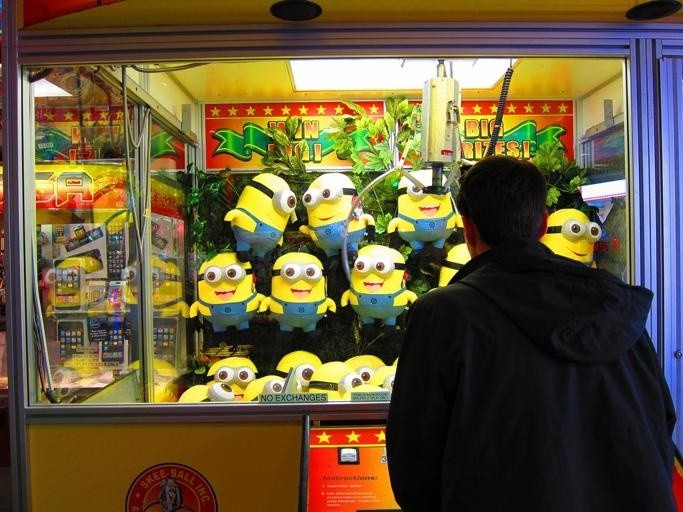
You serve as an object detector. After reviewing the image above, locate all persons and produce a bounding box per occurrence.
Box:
[385,156,676,512]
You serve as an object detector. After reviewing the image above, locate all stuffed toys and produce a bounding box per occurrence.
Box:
[539,208,602,269]
[177,172,471,344]
[177,172,602,404]
[177,351,400,403]
[42,174,189,404]
